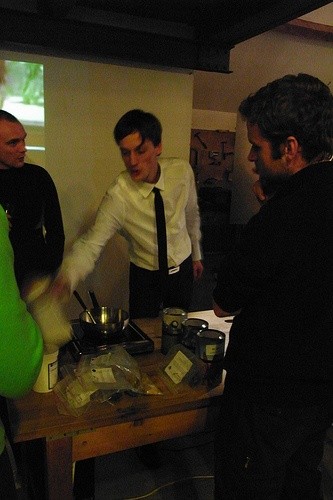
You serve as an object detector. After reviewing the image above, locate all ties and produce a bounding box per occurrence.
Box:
[152,187,167,277]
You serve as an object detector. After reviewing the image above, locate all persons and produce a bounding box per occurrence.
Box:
[0,109,64,306]
[47,110,205,319]
[0,207,44,500]
[211,73,333,500]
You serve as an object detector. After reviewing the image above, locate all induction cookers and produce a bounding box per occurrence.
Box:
[70,317,155,361]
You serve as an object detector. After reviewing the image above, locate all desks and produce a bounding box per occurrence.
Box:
[6,318,224,500]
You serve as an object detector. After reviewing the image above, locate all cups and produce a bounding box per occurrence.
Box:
[31,343,59,393]
[160,307,226,388]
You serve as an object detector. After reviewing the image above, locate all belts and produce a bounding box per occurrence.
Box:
[167,259,188,275]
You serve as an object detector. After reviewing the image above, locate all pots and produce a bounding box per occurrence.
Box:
[79,290,131,336]
